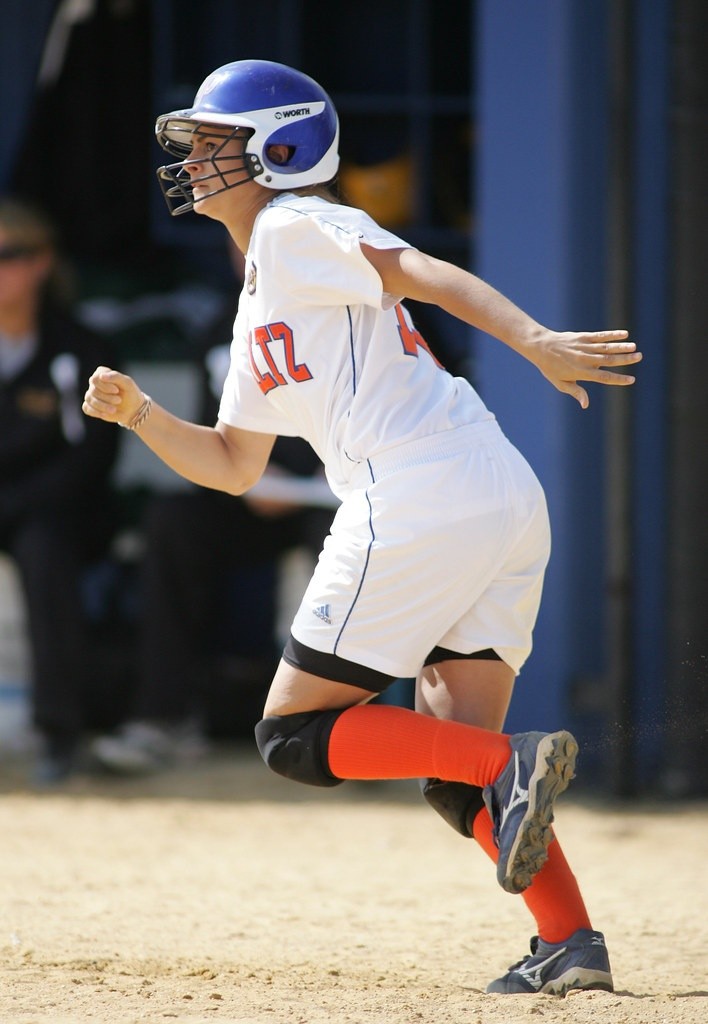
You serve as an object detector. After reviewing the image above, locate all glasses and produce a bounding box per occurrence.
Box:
[0,242,52,264]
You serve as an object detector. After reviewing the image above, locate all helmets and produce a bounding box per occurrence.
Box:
[156,58,343,216]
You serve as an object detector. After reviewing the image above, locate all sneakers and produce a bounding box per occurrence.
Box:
[487,929,614,997]
[481,731,580,893]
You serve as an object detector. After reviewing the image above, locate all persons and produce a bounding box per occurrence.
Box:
[81,59,643,995]
[0,191,127,776]
[242,111,465,652]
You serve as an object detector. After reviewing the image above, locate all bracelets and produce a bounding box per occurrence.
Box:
[118,391,153,431]
